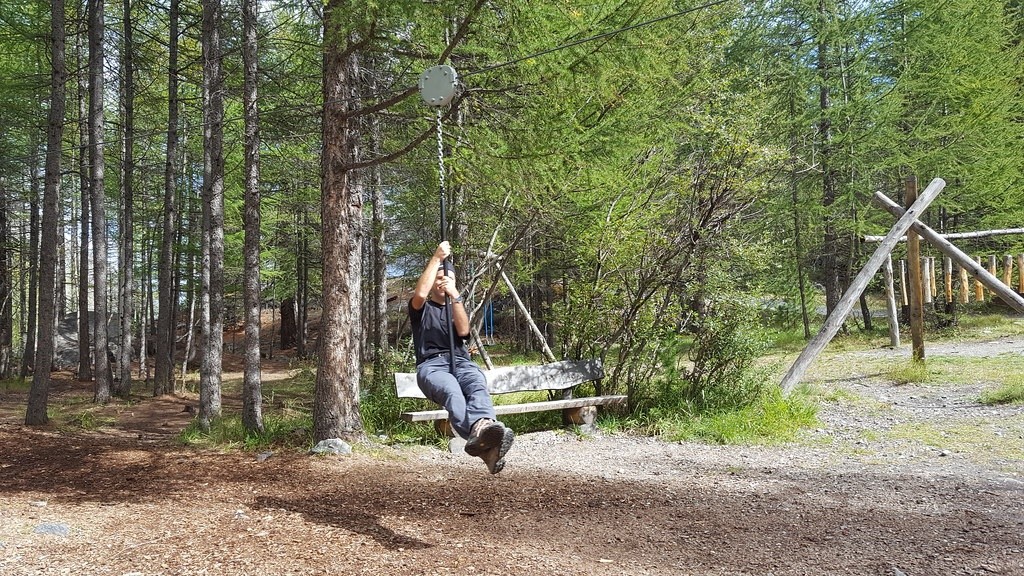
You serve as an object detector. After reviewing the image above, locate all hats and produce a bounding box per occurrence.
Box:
[438,261,457,274]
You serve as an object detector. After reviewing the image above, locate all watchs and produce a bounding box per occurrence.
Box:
[452,295,463,304]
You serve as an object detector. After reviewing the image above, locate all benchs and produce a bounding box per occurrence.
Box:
[394,359,629,438]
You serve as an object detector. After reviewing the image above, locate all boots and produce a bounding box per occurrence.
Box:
[477,428,514,474]
[465,418,505,457]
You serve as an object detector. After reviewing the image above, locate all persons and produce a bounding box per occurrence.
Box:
[407,239,514,473]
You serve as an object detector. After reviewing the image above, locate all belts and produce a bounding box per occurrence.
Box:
[428,354,442,360]
